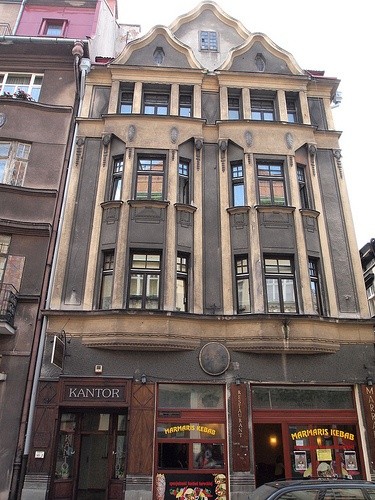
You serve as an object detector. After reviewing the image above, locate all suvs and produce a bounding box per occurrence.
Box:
[244,477,375,500]
